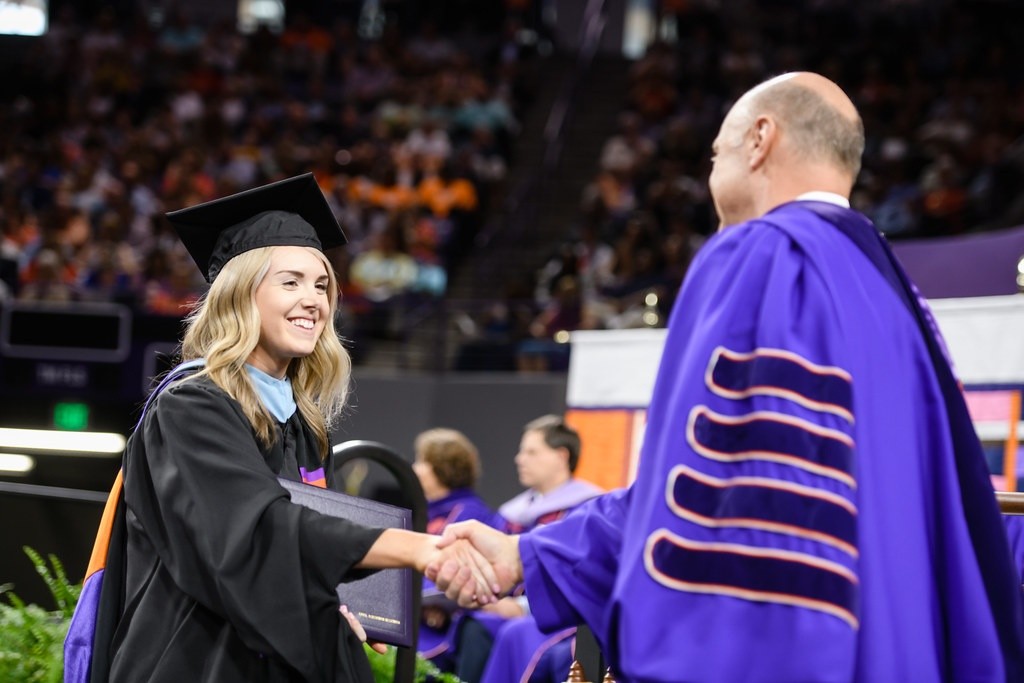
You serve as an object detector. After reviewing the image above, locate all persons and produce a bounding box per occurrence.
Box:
[0,0,1024,372]
[59,173,503,683]
[453,415,604,683]
[425,73,1024,683]
[410,426,496,670]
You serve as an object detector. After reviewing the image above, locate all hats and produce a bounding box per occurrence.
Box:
[167,174,348,282]
